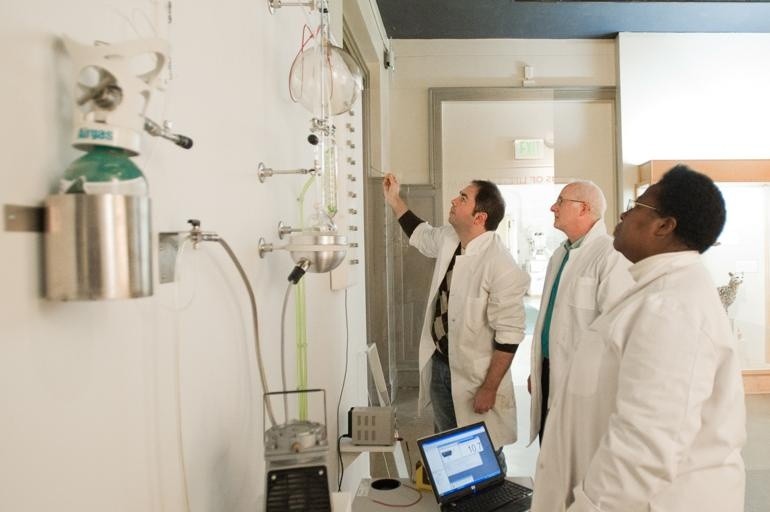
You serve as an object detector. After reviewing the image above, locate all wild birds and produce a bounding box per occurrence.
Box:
[416,421,533,512]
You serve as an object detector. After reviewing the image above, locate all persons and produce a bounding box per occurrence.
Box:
[382,173,531,477]
[529,165,746,511]
[530,180,629,453]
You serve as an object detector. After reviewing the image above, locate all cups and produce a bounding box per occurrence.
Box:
[556,196,583,206]
[627,198,661,212]
